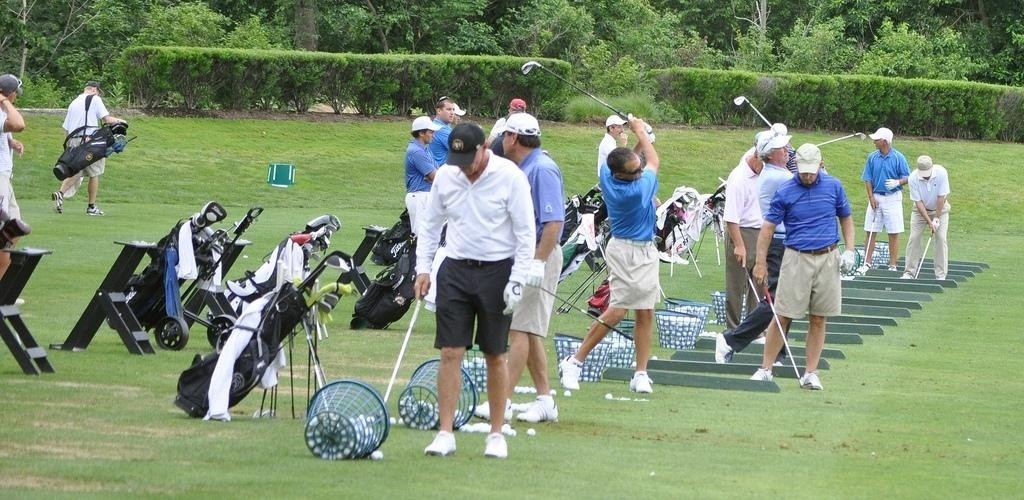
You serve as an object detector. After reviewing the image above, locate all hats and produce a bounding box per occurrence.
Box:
[605,114,628,128]
[451,102,467,117]
[497,113,542,138]
[410,116,442,132]
[84,79,105,94]
[754,123,822,174]
[869,128,894,141]
[509,98,527,111]
[916,155,933,177]
[0,72,24,96]
[445,121,486,166]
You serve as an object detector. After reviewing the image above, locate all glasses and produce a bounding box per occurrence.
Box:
[624,161,643,175]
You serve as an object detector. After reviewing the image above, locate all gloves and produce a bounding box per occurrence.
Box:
[932,218,941,231]
[526,260,546,287]
[500,281,523,317]
[839,250,856,271]
[885,178,900,190]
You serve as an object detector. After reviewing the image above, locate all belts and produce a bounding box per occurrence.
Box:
[451,259,496,268]
[874,191,893,195]
[787,243,838,255]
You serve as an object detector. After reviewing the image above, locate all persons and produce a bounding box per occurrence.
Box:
[0,75,25,305]
[900,156,950,280]
[468,111,565,422]
[562,117,660,393]
[715,124,826,365]
[414,123,536,457]
[861,128,910,270]
[598,115,627,176]
[404,98,466,236]
[487,98,526,157]
[52,81,127,216]
[750,143,854,391]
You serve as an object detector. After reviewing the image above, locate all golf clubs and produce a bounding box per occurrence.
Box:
[193,212,217,230]
[816,132,866,145]
[764,284,813,388]
[671,177,727,281]
[734,95,798,153]
[375,282,432,423]
[521,61,652,135]
[855,206,877,277]
[557,195,610,313]
[252,214,354,420]
[909,230,935,280]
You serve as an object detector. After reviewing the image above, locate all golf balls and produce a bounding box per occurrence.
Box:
[526,429,536,436]
[605,393,611,399]
[552,319,657,382]
[513,386,570,395]
[655,291,749,352]
[389,396,515,436]
[304,410,385,460]
[839,242,891,279]
[460,357,487,393]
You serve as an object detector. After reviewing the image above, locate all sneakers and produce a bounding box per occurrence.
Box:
[715,332,733,366]
[751,368,775,382]
[888,264,897,271]
[86,205,105,216]
[798,372,824,391]
[51,191,64,214]
[484,432,508,459]
[469,398,516,422]
[558,356,583,391]
[899,273,915,279]
[423,429,457,456]
[856,263,873,275]
[629,371,654,394]
[517,394,559,423]
